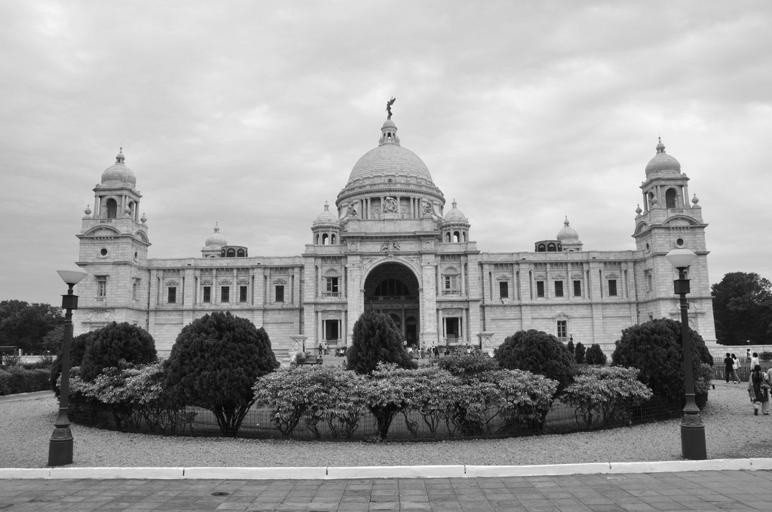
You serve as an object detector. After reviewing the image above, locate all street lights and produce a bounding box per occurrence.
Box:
[46,268,89,468]
[665,247,707,460]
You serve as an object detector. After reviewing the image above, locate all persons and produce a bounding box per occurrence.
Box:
[319,342,322,354]
[731,354,742,384]
[568,337,574,353]
[323,342,328,355]
[746,348,772,415]
[335,347,345,357]
[403,337,474,359]
[724,352,736,384]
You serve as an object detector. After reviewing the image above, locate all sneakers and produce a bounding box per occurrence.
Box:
[754,408,769,415]
[723,380,741,384]
